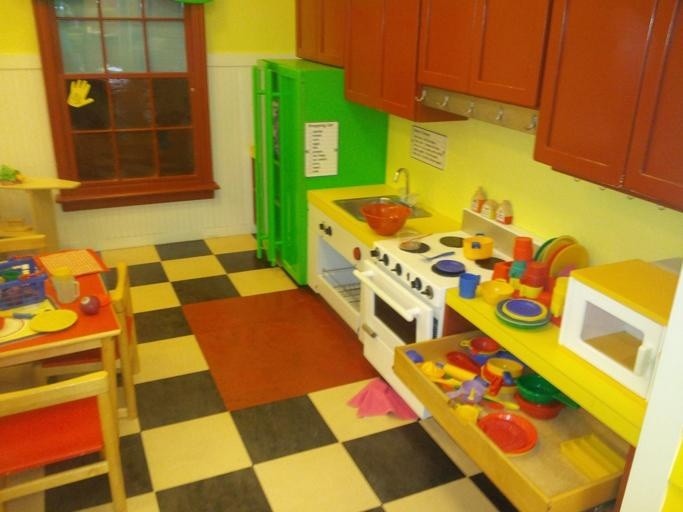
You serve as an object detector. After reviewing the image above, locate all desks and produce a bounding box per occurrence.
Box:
[0,248,122,444]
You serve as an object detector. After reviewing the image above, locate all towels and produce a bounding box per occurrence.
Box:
[348,377,419,421]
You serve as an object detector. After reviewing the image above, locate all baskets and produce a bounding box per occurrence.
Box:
[0,257,48,310]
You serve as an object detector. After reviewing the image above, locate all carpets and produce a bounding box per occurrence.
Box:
[181,288,379,412]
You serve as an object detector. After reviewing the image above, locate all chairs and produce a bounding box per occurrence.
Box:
[31,260,139,418]
[0,370,129,512]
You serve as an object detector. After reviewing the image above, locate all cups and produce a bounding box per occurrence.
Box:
[54,266,80,304]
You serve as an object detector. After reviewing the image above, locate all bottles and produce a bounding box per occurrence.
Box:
[471,186,512,224]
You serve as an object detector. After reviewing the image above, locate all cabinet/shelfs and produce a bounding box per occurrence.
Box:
[251,56,387,285]
[0,173,81,256]
[416,0,551,110]
[391,259,683,511]
[295,0,346,68]
[532,1,682,214]
[346,0,469,122]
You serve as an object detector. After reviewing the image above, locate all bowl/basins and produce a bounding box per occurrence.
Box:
[359,203,411,236]
